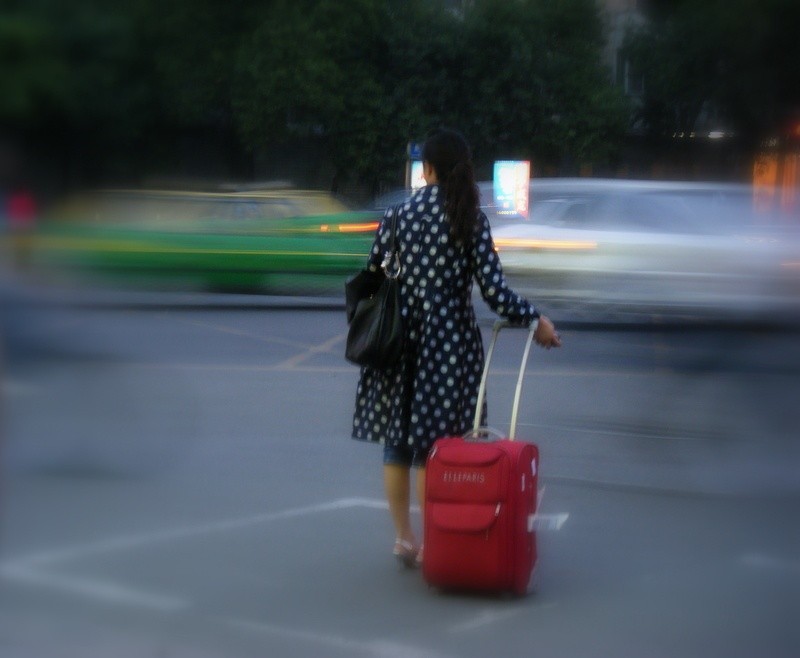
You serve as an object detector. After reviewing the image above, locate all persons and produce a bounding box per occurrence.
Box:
[344,129,562,579]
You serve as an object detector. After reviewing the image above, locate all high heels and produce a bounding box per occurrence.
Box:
[393,540,422,572]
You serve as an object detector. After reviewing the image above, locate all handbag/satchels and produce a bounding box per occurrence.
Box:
[346,202,406,371]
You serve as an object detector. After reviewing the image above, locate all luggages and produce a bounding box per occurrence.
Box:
[423,317,540,597]
